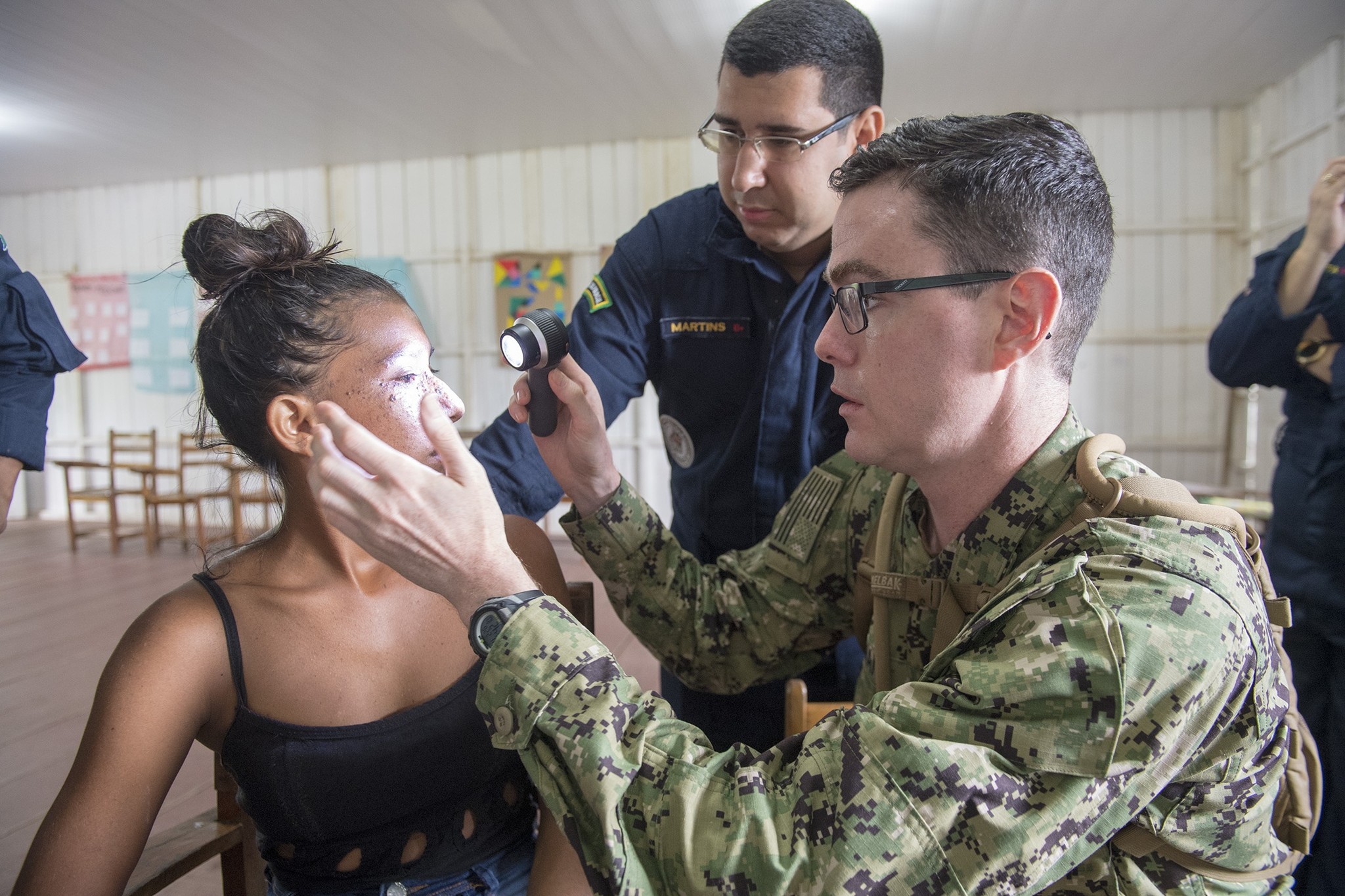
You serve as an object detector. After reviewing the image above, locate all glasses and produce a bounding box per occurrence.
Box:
[831,270,1054,335]
[698,110,864,164]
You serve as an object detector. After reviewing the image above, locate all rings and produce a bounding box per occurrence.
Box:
[1321,174,1336,184]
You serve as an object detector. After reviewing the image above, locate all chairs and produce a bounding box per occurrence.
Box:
[219,458,286,553]
[130,429,239,560]
[52,428,159,558]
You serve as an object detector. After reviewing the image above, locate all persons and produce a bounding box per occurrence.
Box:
[11,206,595,896]
[471,0,884,752]
[0,236,90,532]
[307,112,1325,896]
[1207,159,1345,896]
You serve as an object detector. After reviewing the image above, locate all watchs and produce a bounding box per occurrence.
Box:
[1297,339,1337,364]
[468,590,544,659]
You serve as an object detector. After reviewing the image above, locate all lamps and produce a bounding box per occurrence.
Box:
[497,305,571,439]
